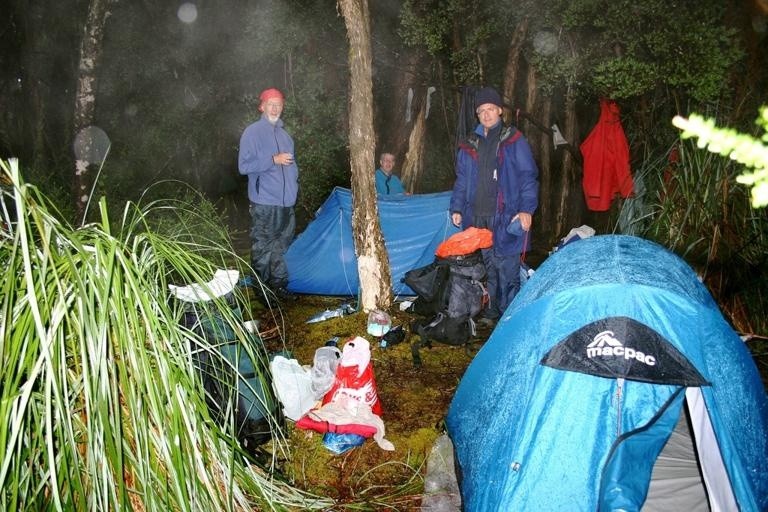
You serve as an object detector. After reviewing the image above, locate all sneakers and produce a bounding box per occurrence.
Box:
[259,287,300,309]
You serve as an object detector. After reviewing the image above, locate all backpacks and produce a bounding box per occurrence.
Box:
[178,311,288,463]
[401,244,492,345]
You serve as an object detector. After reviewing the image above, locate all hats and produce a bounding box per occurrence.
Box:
[474,86,502,110]
[256,89,283,112]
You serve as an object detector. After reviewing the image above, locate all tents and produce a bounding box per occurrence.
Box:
[244,185,462,297]
[443,233,767,511]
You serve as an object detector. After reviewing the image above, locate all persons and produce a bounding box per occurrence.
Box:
[376,150,411,195]
[450,86,540,315]
[238,89,298,308]
